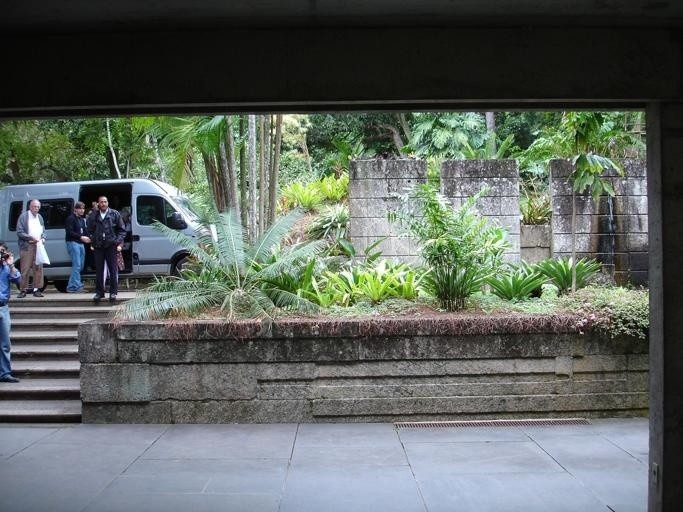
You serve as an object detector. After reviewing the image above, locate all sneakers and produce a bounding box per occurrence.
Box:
[66,287,89,293]
[33,291,44,297]
[17,291,26,298]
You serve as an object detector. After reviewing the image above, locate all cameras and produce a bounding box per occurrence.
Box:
[0,249,9,262]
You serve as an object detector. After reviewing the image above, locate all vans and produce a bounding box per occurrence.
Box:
[0,178,216,294]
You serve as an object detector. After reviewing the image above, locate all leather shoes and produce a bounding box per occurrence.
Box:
[94,292,104,300]
[110,293,116,300]
[0,374,19,382]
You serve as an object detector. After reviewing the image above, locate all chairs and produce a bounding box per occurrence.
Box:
[46,205,64,226]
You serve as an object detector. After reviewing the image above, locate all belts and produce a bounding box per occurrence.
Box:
[0,301,7,307]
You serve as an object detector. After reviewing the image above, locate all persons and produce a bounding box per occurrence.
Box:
[64,194,131,301]
[15,199,46,298]
[0,239,19,383]
